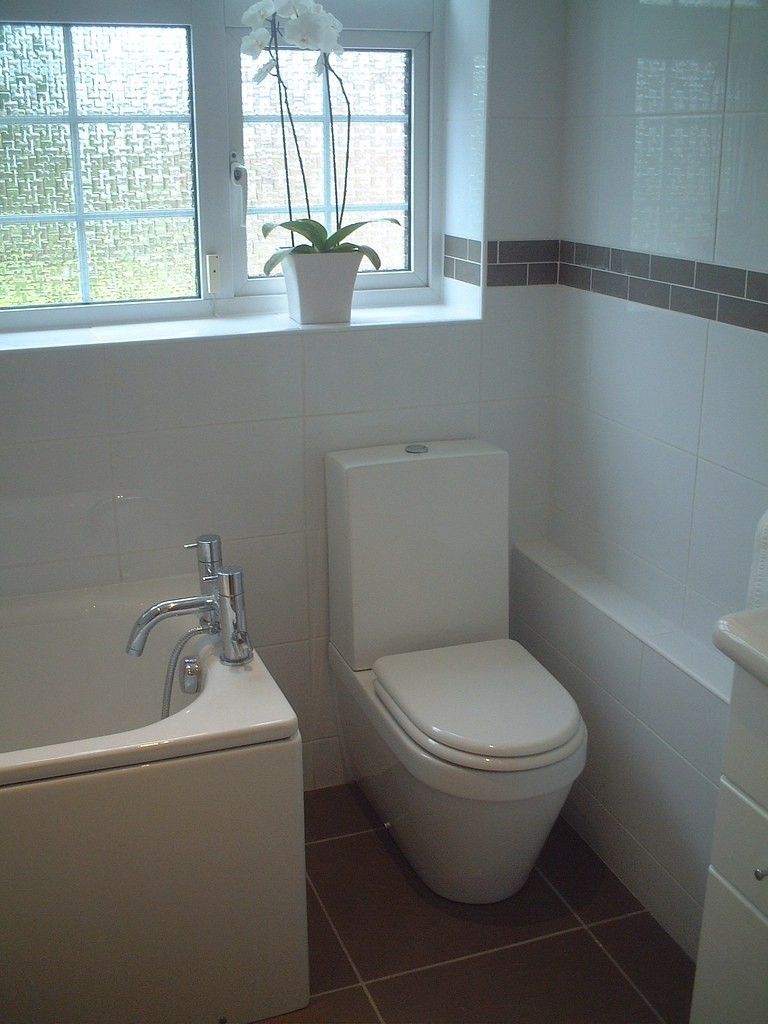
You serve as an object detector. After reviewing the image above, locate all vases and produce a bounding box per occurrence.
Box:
[274,245,365,326]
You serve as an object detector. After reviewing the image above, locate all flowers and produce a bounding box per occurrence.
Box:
[240,1,402,276]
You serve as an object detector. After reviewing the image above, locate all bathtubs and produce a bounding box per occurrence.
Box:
[0,572,309,1022]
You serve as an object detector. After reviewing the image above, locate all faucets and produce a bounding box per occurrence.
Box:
[125,596,219,658]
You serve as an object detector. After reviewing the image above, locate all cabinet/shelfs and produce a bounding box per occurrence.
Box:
[689,608,768,1024]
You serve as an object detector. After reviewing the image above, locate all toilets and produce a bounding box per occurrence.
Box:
[325,434,589,905]
